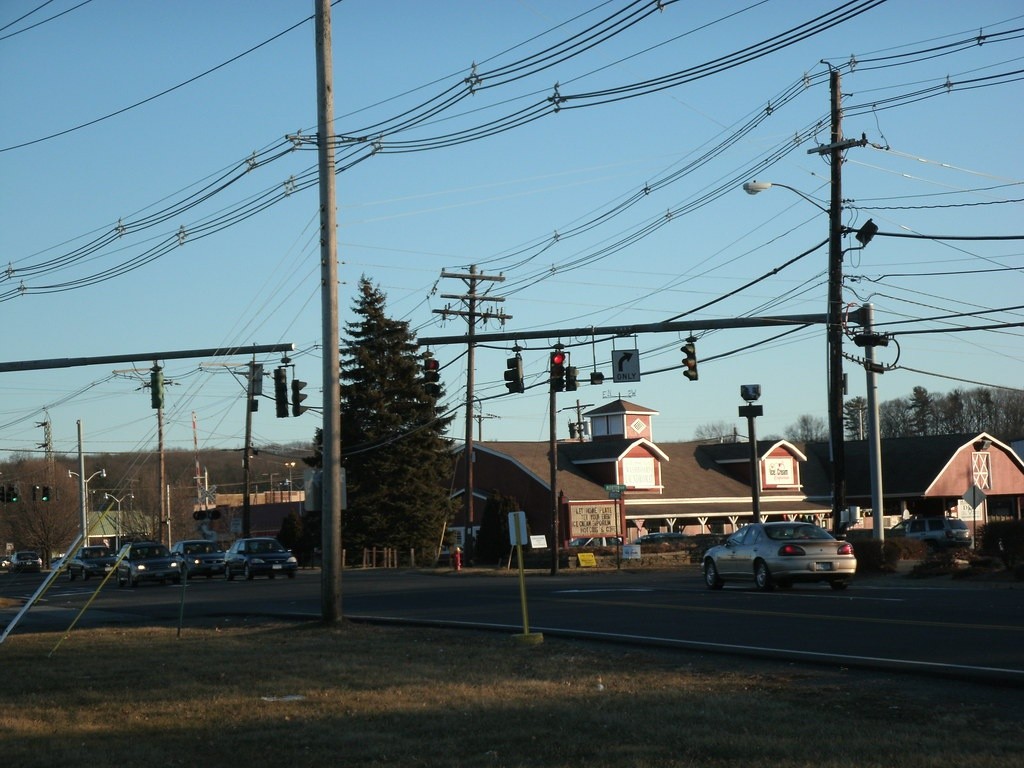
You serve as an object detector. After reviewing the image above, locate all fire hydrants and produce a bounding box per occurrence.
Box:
[450,547,462,572]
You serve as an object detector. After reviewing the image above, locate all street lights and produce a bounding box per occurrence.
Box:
[103,491,135,552]
[285,462,296,503]
[67,468,107,546]
[741,72,845,545]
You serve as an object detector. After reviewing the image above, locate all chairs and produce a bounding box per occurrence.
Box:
[257,543,266,553]
[146,547,156,558]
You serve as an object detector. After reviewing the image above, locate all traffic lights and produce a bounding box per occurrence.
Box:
[680,342,699,380]
[504,354,524,394]
[0,483,20,502]
[549,351,565,394]
[290,380,309,418]
[42,486,51,503]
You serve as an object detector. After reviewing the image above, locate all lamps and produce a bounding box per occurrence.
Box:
[979,440,992,450]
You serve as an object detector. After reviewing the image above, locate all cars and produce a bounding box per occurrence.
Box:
[565,516,971,551]
[701,521,856,593]
[8,537,299,587]
